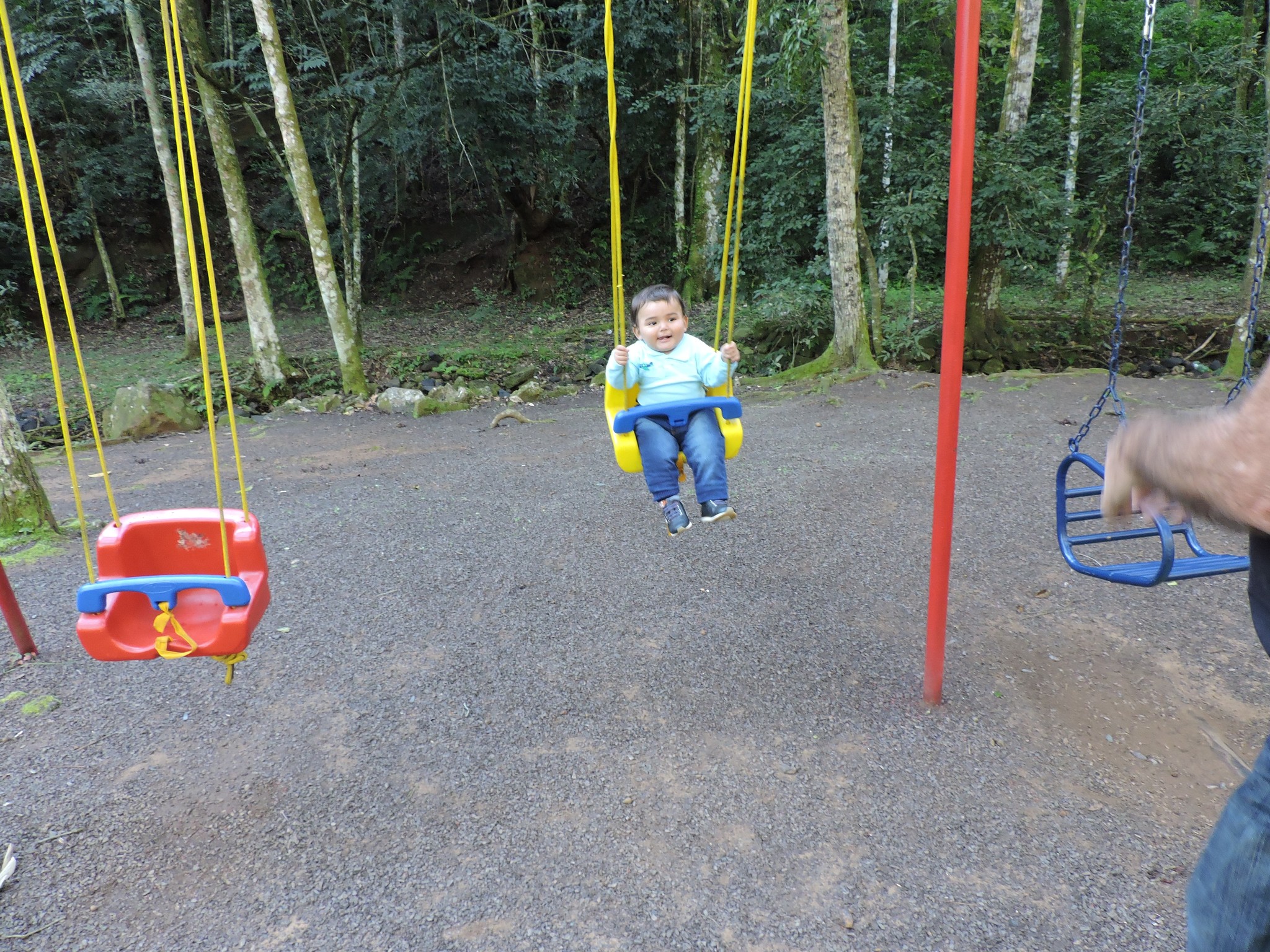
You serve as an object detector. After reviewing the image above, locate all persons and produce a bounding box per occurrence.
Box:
[1100,355,1270,952]
[605,283,741,537]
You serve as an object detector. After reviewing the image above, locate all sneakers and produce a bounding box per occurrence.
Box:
[663,500,692,537]
[700,500,737,522]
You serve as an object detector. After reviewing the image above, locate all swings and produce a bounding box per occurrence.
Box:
[1057,0,1269,587]
[0,0,270,682]
[605,0,757,484]
